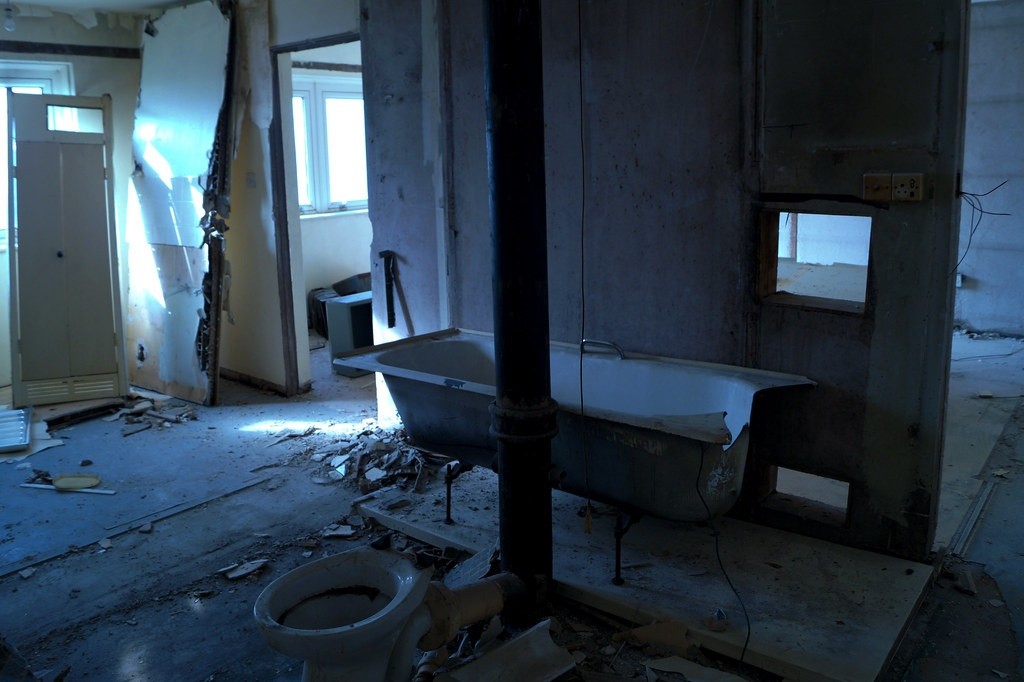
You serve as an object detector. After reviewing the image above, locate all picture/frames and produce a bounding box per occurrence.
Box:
[863,174,891,201]
[891,173,922,202]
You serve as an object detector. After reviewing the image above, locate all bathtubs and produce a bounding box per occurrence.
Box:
[332,324,820,527]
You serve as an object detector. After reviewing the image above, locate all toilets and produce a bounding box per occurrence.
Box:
[253,530,450,682]
